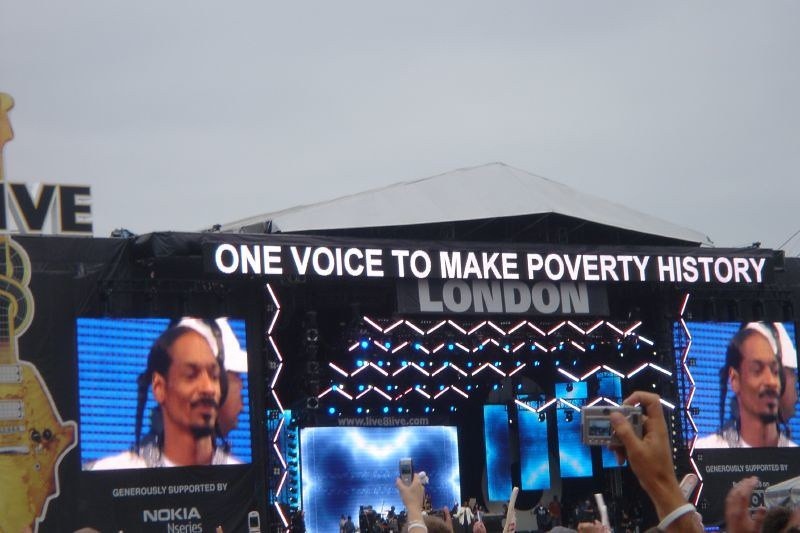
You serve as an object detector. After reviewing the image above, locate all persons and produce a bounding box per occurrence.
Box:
[739,321,786,397]
[359,391,800,533]
[343,515,355,533]
[294,510,305,533]
[83,326,242,466]
[214,317,247,444]
[339,514,345,533]
[686,328,798,448]
[774,322,799,423]
[168,316,227,411]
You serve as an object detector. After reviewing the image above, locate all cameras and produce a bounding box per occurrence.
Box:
[249,511,260,533]
[580,403,644,448]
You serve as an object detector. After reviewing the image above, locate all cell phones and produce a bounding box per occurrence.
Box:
[399,457,413,487]
